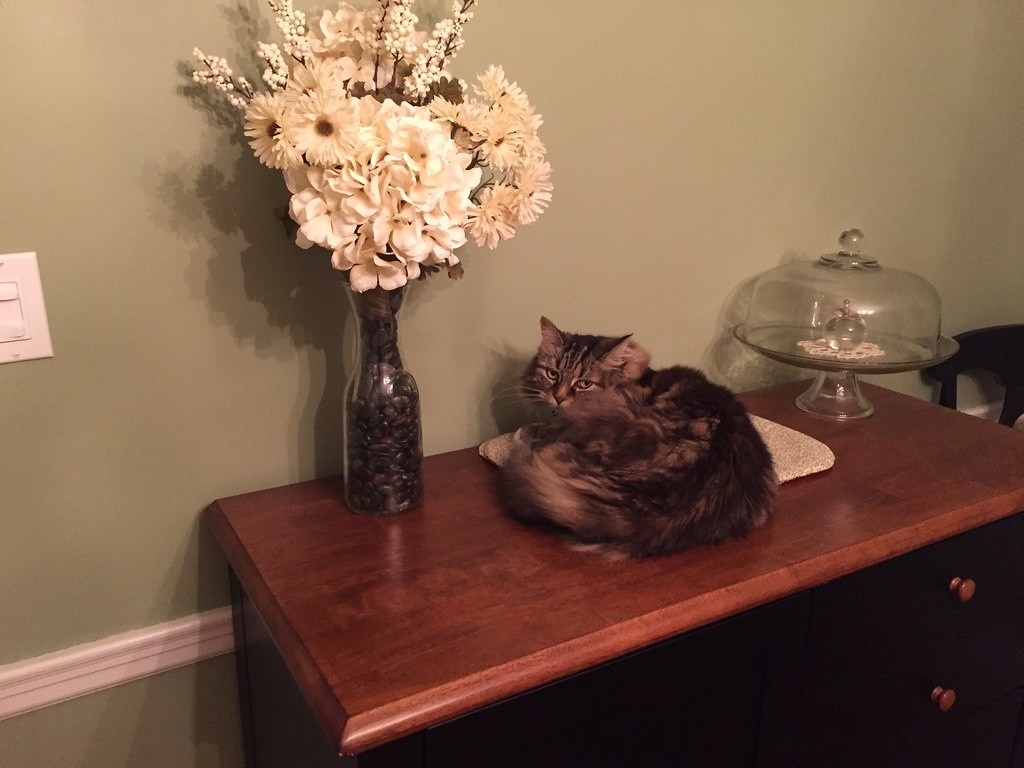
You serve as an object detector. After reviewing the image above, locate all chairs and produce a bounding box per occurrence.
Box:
[921,322,1024,429]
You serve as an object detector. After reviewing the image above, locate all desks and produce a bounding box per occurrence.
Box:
[206,375,1024,766]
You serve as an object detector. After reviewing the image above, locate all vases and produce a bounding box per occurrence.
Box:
[333,265,421,516]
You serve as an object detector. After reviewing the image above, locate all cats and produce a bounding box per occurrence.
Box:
[497,314,785,560]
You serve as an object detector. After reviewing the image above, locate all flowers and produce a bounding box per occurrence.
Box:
[188,0,553,295]
